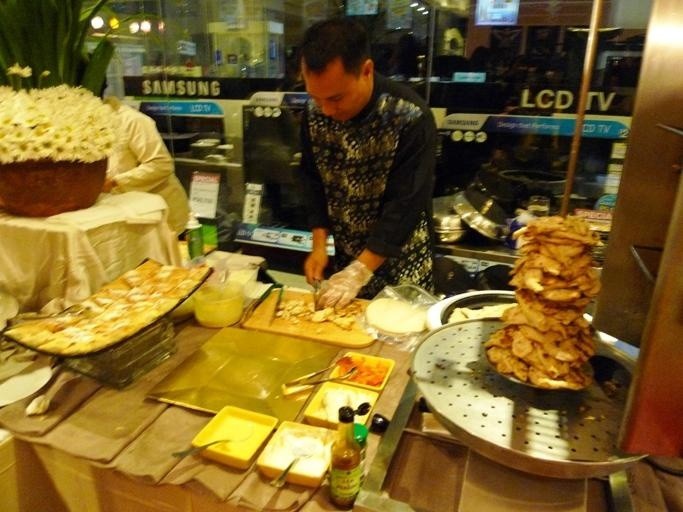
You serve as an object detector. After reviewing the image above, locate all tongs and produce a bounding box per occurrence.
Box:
[278,355,360,396]
[241,283,287,328]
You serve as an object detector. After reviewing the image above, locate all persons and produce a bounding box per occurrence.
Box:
[287,19,437,310]
[94,93,191,241]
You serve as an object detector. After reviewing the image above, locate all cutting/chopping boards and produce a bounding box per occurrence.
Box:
[241,289,380,349]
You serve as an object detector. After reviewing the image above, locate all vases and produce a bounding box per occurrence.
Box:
[0,163,110,216]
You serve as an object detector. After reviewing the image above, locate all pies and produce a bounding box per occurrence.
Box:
[5,260,209,355]
[276,296,362,330]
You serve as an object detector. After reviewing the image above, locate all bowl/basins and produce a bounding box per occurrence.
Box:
[188,138,235,160]
[189,282,244,328]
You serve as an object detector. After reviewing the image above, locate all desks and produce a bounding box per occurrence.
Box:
[0,192,181,310]
[0,266,607,512]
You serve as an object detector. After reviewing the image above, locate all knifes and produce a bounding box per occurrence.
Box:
[312,277,319,312]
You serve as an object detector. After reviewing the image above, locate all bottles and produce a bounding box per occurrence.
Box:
[183,210,206,261]
[329,403,363,511]
[349,422,368,487]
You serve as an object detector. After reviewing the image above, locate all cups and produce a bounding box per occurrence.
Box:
[528,195,550,218]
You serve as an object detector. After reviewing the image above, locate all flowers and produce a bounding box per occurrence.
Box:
[0,63,117,166]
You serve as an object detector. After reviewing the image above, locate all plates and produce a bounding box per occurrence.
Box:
[328,349,396,393]
[190,404,279,472]
[1,253,216,361]
[303,379,381,429]
[259,417,338,489]
[0,357,55,408]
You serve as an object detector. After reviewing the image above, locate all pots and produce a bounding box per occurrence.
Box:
[430,189,510,250]
